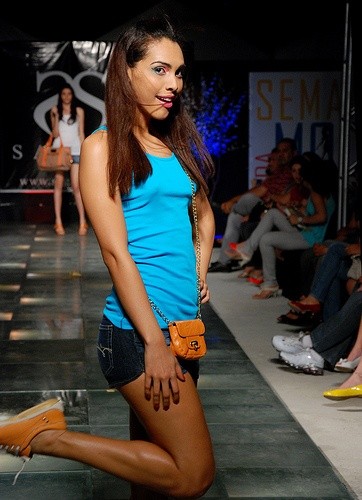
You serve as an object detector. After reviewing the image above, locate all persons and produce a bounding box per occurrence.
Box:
[1,19,215,496]
[198,137,361,400]
[49,83,88,235]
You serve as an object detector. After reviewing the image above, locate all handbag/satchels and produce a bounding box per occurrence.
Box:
[168,318,208,360]
[33,130,73,172]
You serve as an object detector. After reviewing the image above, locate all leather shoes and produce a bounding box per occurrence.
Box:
[323,384,362,401]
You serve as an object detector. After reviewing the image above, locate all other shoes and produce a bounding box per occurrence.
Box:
[206,263,233,272]
[54,223,65,235]
[78,223,88,236]
[0,397,68,459]
[209,260,239,267]
[246,276,264,286]
[251,285,279,300]
[237,268,252,278]
[222,249,242,260]
[272,330,312,355]
[279,350,325,376]
[334,356,362,372]
[227,242,252,266]
[276,310,318,326]
[287,300,321,316]
